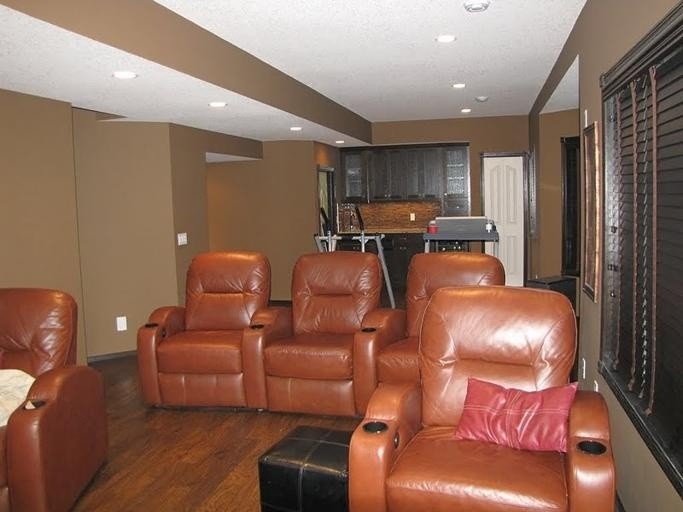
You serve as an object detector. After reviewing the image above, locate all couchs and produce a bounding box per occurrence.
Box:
[355,251,506,415]
[263,251,405,417]
[136,251,270,418]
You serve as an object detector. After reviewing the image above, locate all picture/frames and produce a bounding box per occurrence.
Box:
[582,121,600,304]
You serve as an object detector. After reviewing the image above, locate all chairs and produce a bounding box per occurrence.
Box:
[0,288,108,512]
[349,285,615,512]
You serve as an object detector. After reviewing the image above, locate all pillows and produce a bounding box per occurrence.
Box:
[452,377,578,453]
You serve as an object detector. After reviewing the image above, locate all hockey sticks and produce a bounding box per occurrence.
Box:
[353,205,365,251]
[319,207,332,252]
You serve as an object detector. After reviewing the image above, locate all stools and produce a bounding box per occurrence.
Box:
[258,425,354,512]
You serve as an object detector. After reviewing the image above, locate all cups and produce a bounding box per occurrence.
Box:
[426,224,439,234]
[483,216,494,234]
